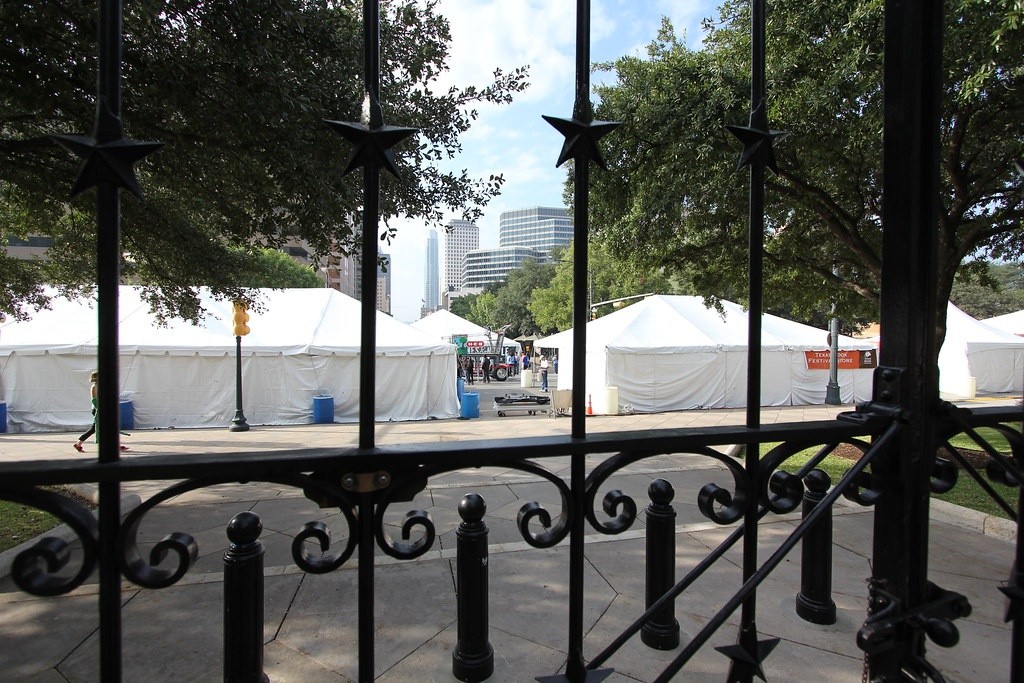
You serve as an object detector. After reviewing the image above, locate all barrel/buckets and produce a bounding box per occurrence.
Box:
[457,379,464,403]
[962,377,975,397]
[461,392,481,418]
[313,394,334,423]
[0,400,7,433]
[520,370,532,388]
[602,386,618,416]
[119,398,134,430]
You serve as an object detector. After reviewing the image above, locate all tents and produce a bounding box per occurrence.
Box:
[939,300,1024,398]
[532,294,878,416]
[409,308,522,354]
[0,285,460,432]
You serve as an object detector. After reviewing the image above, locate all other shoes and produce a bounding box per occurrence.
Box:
[120,446,128,450]
[74,444,85,452]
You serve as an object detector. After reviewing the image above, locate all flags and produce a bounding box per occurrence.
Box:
[499,325,510,334]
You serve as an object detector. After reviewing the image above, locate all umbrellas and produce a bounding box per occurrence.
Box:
[515,333,546,340]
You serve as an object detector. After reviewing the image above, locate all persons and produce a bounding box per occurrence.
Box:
[506,350,531,378]
[465,359,474,385]
[72,372,130,451]
[551,354,558,374]
[537,355,550,392]
[482,355,491,383]
